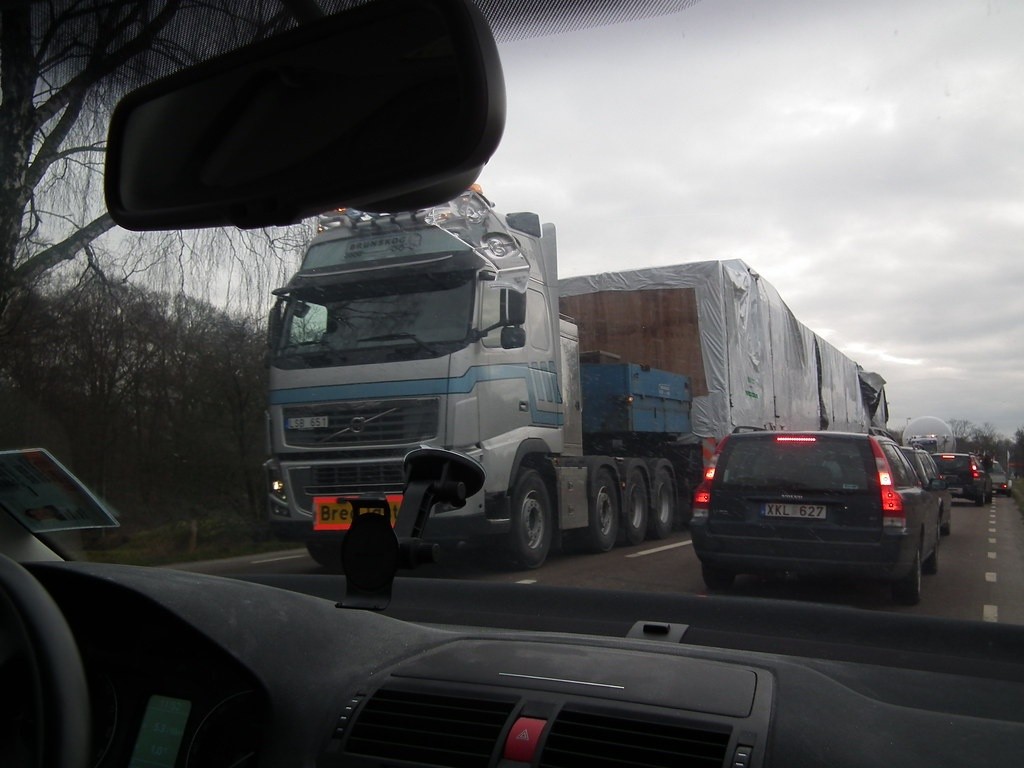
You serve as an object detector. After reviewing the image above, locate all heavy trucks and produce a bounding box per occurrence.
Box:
[261,188,891,579]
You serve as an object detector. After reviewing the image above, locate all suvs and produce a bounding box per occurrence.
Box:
[690,425,950,605]
[927,451,993,509]
[897,444,960,536]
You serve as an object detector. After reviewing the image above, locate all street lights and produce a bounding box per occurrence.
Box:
[906,417,911,426]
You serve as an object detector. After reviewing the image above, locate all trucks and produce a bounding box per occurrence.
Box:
[907,434,940,453]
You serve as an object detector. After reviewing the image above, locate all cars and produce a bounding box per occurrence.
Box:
[978,459,1014,497]
